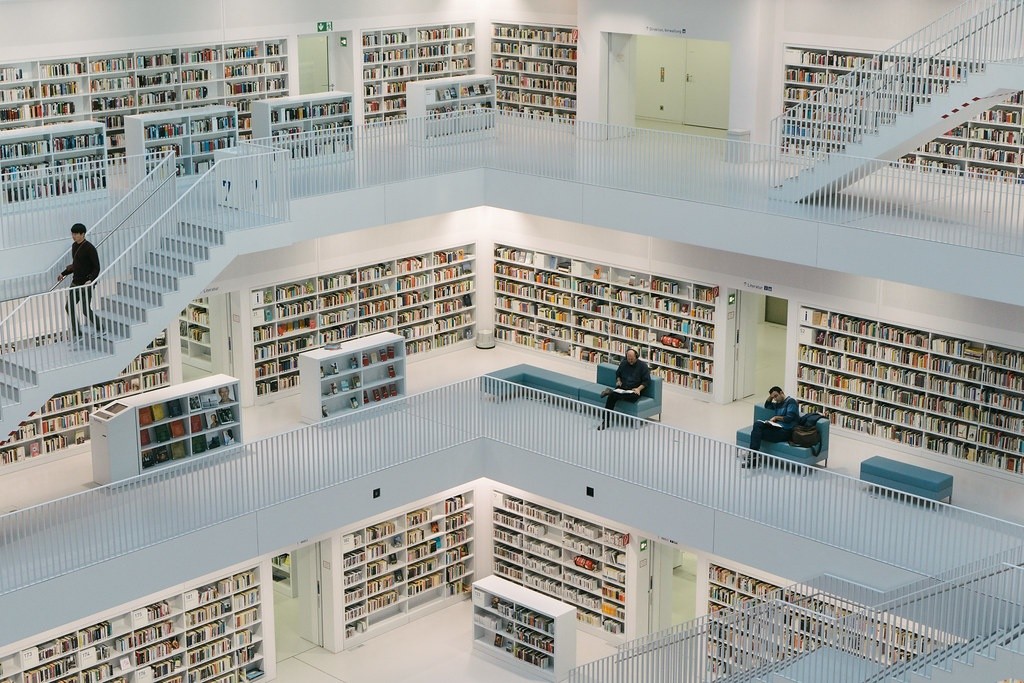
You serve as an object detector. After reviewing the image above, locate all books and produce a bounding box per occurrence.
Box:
[795,307,1024,478]
[491,23,577,125]
[271,491,626,669]
[0,571,264,683]
[756,418,784,428]
[0,42,290,104]
[0,290,240,472]
[0,102,355,203]
[781,47,1024,184]
[705,562,935,683]
[613,388,634,394]
[252,249,476,416]
[363,25,492,127]
[495,247,720,395]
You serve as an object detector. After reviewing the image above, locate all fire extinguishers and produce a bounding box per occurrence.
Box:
[660,335,686,349]
[574,555,599,573]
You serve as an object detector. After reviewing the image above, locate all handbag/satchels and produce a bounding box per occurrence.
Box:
[792,425,822,457]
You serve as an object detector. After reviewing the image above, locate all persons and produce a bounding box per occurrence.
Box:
[210,414,219,427]
[189,397,196,409]
[227,428,235,445]
[597,349,651,430]
[742,388,799,470]
[218,386,233,403]
[194,396,200,408]
[58,223,107,344]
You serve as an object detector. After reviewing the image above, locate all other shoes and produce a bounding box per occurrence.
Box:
[598,420,609,430]
[91,330,106,339]
[600,388,613,398]
[67,335,82,347]
[742,455,760,467]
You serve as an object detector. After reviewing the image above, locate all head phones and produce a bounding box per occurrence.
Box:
[626,349,639,359]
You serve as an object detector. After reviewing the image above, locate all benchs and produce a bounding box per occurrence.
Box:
[736,402,830,478]
[579,362,662,431]
[480,364,595,412]
[859,455,954,511]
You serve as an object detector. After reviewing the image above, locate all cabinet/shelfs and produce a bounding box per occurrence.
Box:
[248,240,478,406]
[250,89,357,175]
[703,559,951,683]
[271,549,299,598]
[0,35,295,216]
[488,19,580,135]
[492,238,720,404]
[775,43,1024,197]
[360,19,479,133]
[179,296,214,373]
[490,486,631,645]
[298,331,407,428]
[0,324,172,477]
[406,73,497,149]
[119,373,244,480]
[340,489,477,650]
[795,301,1024,486]
[0,561,267,683]
[471,575,578,683]
